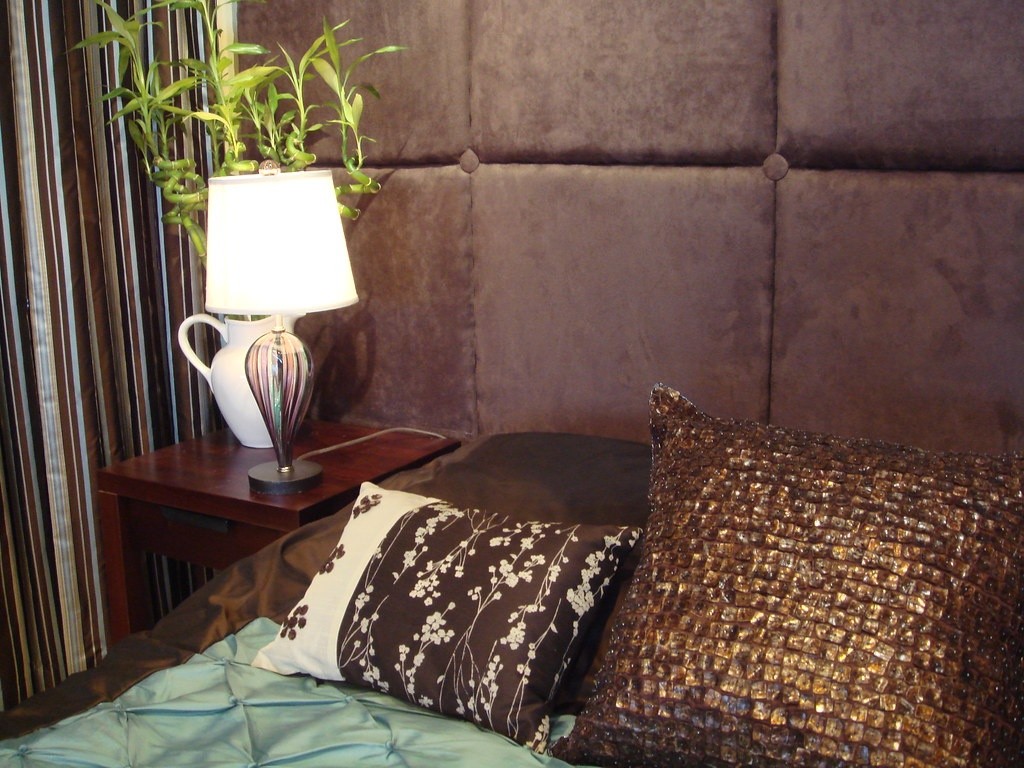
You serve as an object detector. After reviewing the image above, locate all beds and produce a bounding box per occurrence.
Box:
[0,430,651,768]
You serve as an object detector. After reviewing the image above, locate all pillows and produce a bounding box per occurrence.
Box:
[546,382,1024,768]
[251,480,645,748]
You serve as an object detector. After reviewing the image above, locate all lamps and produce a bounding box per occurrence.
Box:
[205,170,362,497]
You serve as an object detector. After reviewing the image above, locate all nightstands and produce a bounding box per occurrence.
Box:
[99,419,462,642]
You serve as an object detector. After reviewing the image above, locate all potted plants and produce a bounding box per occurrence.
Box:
[65,0,409,452]
